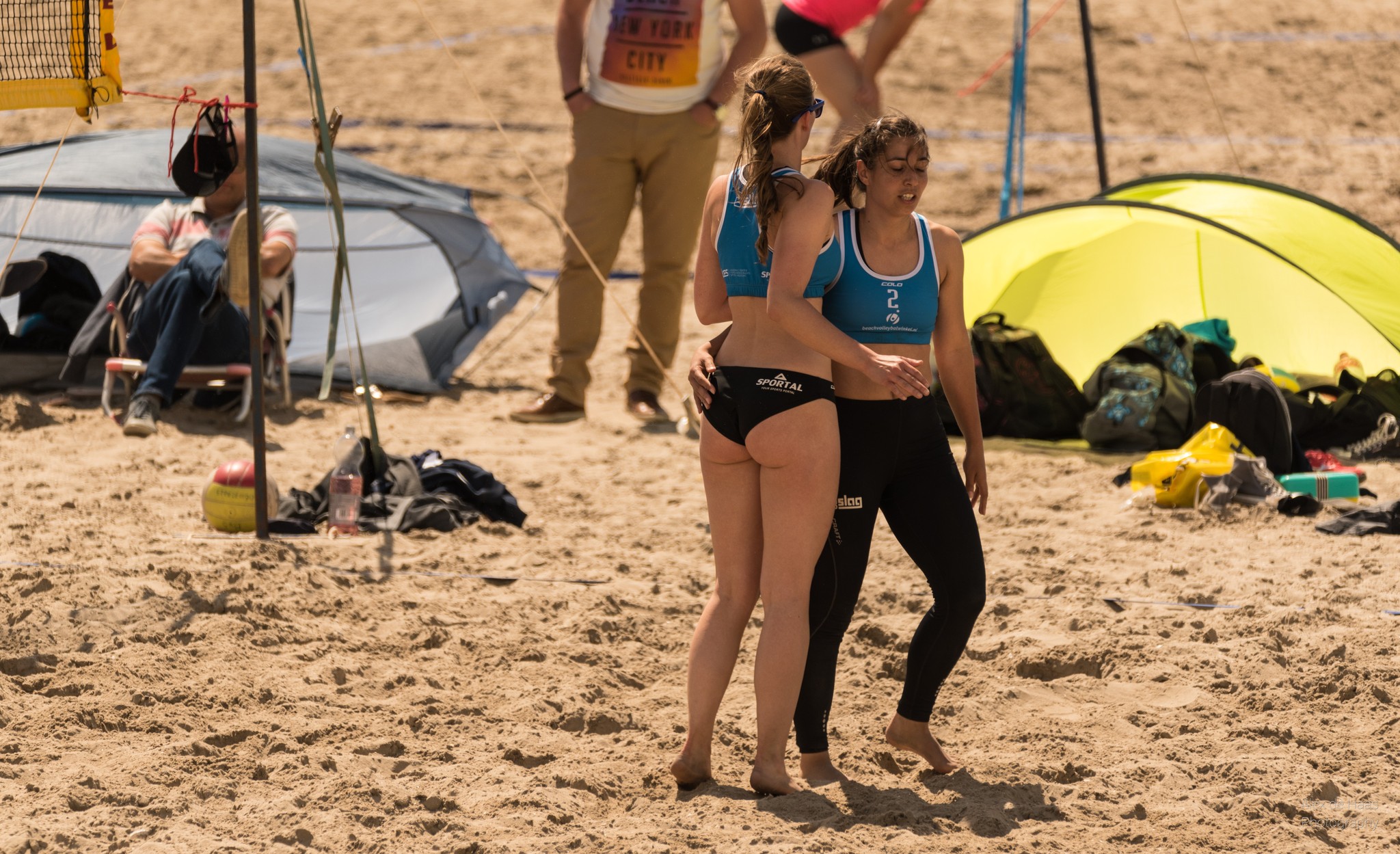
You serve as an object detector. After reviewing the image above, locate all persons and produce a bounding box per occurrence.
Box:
[669,55,989,796]
[122,124,299,436]
[772,0,928,159]
[511,0,768,421]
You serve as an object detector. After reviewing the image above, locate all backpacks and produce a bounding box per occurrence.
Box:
[967,313,1089,440]
[1081,320,1399,472]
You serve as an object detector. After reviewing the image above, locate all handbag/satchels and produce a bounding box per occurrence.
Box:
[1130,421,1255,507]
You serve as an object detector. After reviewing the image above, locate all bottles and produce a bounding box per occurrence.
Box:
[1275,472,1359,501]
[328,426,364,536]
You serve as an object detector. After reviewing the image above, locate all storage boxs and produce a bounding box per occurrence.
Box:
[1278,472,1360,505]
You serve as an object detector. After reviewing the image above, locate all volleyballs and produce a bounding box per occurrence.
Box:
[201,459,279,534]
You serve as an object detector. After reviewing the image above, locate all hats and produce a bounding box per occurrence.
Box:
[172,103,238,197]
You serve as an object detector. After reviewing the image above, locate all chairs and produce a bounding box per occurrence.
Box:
[100,275,294,424]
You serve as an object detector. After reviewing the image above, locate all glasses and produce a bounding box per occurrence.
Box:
[792,98,826,124]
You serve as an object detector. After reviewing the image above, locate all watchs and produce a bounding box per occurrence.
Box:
[704,96,729,124]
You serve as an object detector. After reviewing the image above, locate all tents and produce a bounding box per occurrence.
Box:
[961,174,1400,406]
[0,126,531,396]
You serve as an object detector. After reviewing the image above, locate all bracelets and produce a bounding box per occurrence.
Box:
[564,87,583,100]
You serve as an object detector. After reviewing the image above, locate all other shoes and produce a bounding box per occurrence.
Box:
[1231,455,1300,503]
[220,210,263,307]
[508,389,585,422]
[125,397,158,434]
[625,388,670,421]
